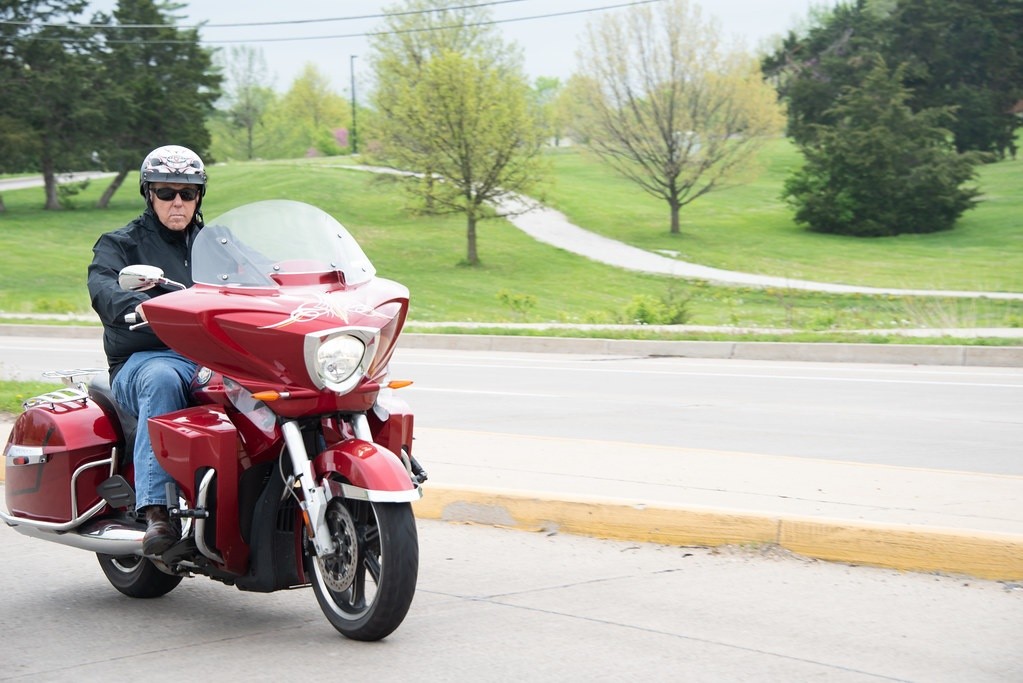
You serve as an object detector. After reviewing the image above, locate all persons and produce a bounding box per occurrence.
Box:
[87,146,276,556]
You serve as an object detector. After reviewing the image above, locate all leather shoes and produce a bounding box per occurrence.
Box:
[142,505,182,556]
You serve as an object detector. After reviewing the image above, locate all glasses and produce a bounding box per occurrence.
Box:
[150,185,202,201]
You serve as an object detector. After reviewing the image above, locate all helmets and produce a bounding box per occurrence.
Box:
[140,145,207,194]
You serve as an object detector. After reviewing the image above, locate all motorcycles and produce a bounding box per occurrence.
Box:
[0,198,428,641]
